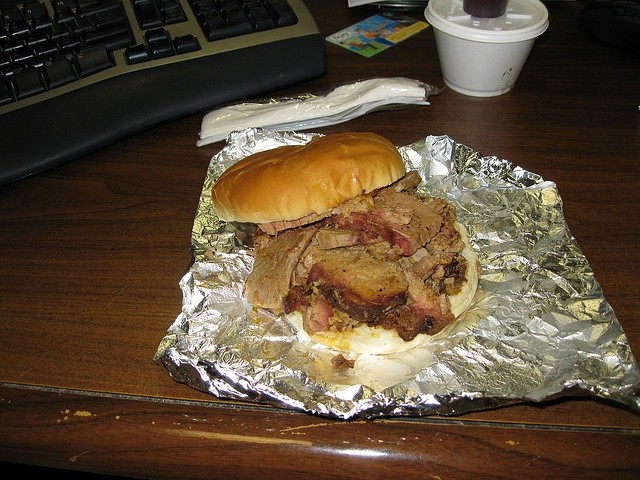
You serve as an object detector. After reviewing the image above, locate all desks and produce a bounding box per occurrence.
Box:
[0,1,639,480]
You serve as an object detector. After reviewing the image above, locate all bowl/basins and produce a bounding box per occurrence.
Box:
[424,0,550,97]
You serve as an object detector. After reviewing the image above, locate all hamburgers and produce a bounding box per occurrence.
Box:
[211,131,481,356]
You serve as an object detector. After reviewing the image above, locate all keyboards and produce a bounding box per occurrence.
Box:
[1,1,328,187]
